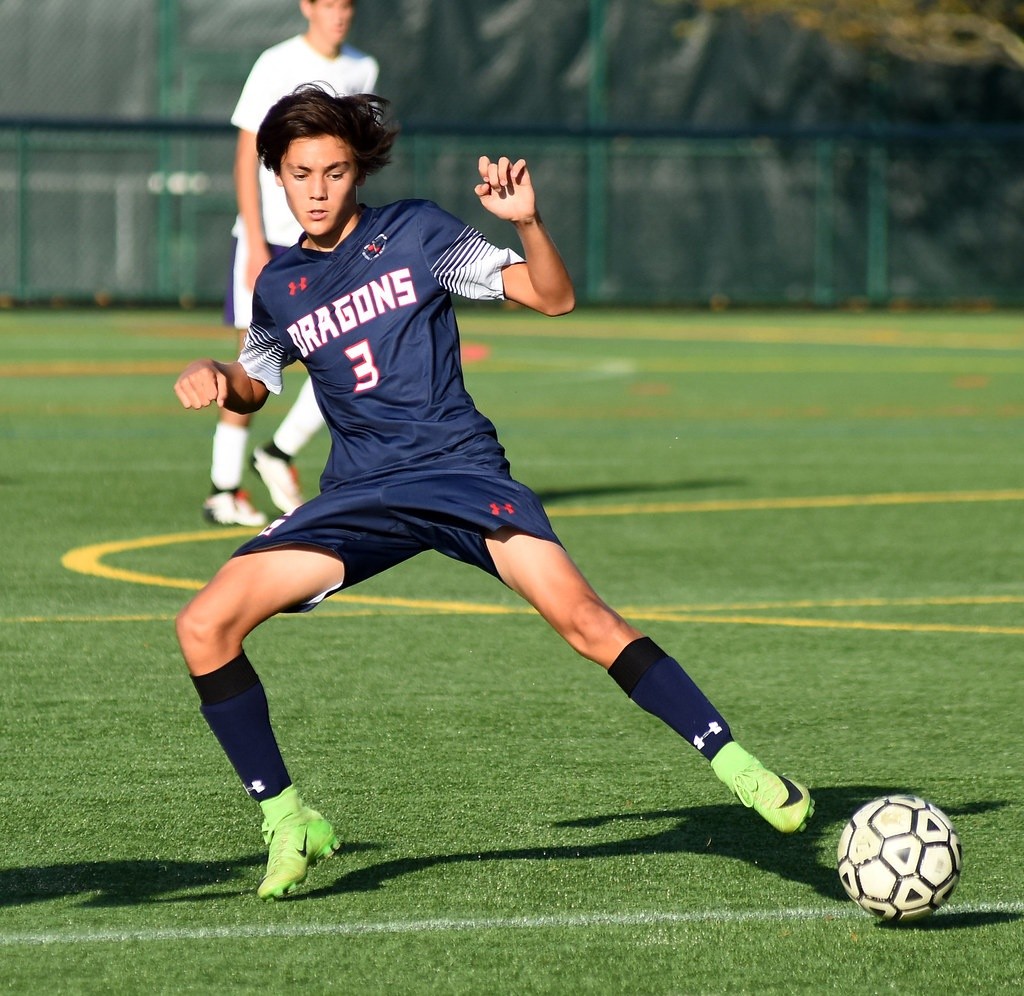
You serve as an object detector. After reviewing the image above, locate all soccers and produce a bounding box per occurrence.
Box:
[833,793,963,921]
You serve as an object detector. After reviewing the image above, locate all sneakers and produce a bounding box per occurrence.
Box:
[709,740,814,836]
[254,785,341,903]
[249,442,301,516]
[200,489,269,529]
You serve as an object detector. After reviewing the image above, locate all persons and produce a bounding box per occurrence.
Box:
[175,77,817,902]
[200,0,381,529]
